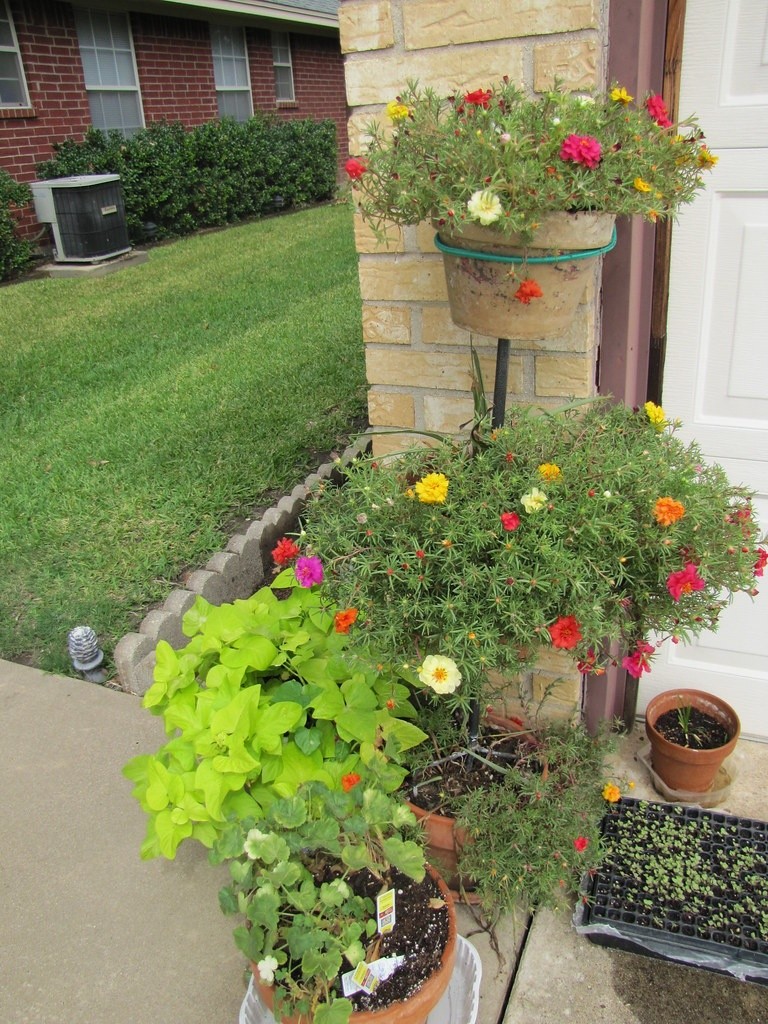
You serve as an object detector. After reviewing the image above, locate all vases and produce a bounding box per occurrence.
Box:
[402,721,550,905]
[426,200,614,342]
[236,853,457,1023]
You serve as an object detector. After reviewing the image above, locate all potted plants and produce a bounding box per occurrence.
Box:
[646,689,742,805]
[573,798,768,983]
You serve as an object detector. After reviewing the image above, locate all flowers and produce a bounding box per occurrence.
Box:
[136,587,426,1023]
[342,71,717,306]
[270,380,767,685]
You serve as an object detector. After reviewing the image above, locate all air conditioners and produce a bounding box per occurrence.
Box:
[33,173,133,264]
